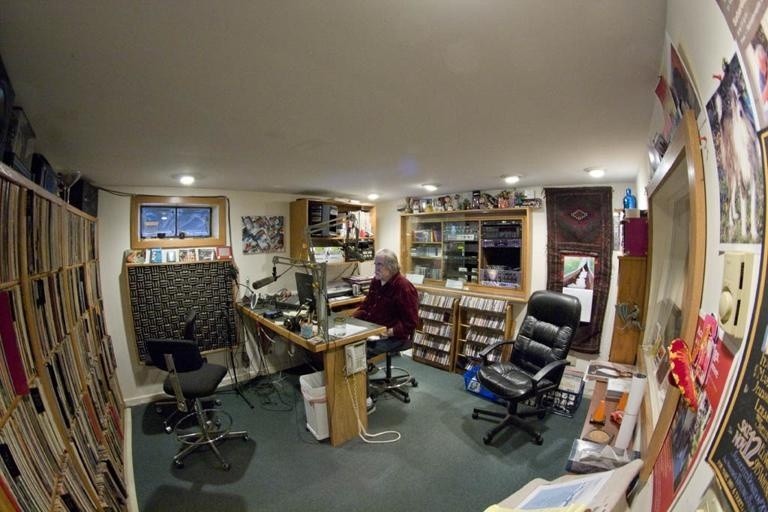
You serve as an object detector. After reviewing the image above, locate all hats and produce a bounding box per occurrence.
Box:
[346,214,357,222]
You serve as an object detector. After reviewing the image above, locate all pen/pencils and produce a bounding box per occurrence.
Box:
[308,310,315,325]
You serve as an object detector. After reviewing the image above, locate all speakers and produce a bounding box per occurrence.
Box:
[69,179,98,218]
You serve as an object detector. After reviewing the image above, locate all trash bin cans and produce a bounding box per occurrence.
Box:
[299,371,330,441]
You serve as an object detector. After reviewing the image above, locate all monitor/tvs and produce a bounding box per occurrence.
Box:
[295,272,331,325]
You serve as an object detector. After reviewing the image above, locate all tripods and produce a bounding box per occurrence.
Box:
[214,300,259,408]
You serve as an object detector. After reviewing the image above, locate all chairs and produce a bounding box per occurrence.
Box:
[472,290,582,445]
[145,336,248,471]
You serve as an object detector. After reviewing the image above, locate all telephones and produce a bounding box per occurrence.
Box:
[344,340,368,377]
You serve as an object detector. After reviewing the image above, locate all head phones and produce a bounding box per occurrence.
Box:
[278,317,300,331]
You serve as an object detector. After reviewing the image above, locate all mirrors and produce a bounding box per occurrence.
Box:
[635,106,708,485]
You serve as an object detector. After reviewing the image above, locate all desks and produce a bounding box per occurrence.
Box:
[236,288,389,448]
[569,362,643,477]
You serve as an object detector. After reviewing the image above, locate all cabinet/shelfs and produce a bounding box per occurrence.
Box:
[0,161,140,512]
[399,206,532,376]
[289,199,378,263]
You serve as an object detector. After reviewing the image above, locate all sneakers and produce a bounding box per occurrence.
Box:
[366,397,376,416]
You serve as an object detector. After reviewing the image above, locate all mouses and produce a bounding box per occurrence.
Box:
[334,317,344,322]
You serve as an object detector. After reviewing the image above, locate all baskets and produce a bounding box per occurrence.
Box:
[464,365,496,401]
[521,375,585,418]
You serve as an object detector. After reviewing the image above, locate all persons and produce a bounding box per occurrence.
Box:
[717,84,762,243]
[753,43,768,108]
[345,247,419,417]
[345,214,359,240]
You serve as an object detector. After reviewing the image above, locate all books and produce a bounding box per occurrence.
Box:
[1,179,131,512]
[412,227,441,281]
[411,289,508,372]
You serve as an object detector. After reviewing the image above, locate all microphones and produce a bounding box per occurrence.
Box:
[347,246,365,262]
[253,277,276,289]
